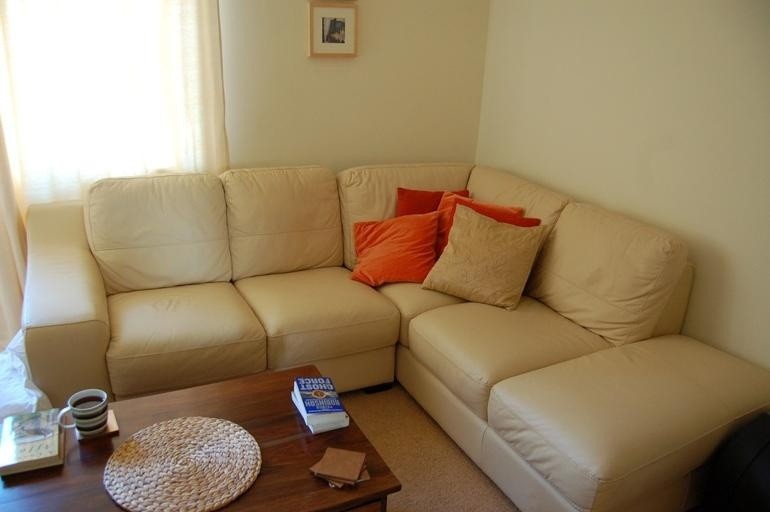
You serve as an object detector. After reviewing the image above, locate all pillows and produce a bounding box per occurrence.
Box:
[350,186,549,312]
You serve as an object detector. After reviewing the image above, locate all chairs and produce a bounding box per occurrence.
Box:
[696,412,770,512]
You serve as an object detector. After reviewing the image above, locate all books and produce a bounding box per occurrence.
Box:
[0,408,66,479]
[290,389,350,436]
[308,445,371,489]
[293,374,345,427]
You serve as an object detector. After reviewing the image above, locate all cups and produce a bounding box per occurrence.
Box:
[56,386,110,438]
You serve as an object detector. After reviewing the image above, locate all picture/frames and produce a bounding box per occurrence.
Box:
[307,2,359,58]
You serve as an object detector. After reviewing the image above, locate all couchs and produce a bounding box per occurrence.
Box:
[22,165,770,511]
